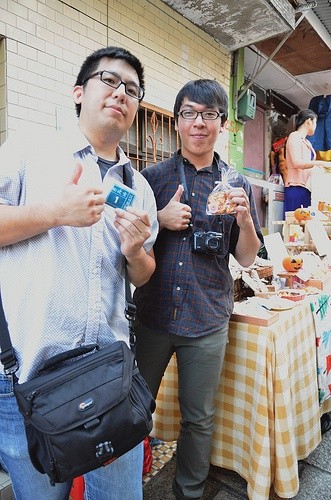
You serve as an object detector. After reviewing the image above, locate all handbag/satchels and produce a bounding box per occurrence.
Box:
[11,340,156,487]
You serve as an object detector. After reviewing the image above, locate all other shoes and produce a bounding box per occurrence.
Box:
[171,484,200,500]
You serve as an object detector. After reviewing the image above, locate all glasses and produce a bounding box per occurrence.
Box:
[177,109,224,120]
[80,70,145,102]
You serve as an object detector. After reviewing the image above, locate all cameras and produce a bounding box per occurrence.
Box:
[192,231,223,255]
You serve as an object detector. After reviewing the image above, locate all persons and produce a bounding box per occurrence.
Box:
[128,79,265,500]
[282,109,330,222]
[0,46,159,500]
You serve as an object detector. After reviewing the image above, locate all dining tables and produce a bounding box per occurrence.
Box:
[147,280,330,500]
